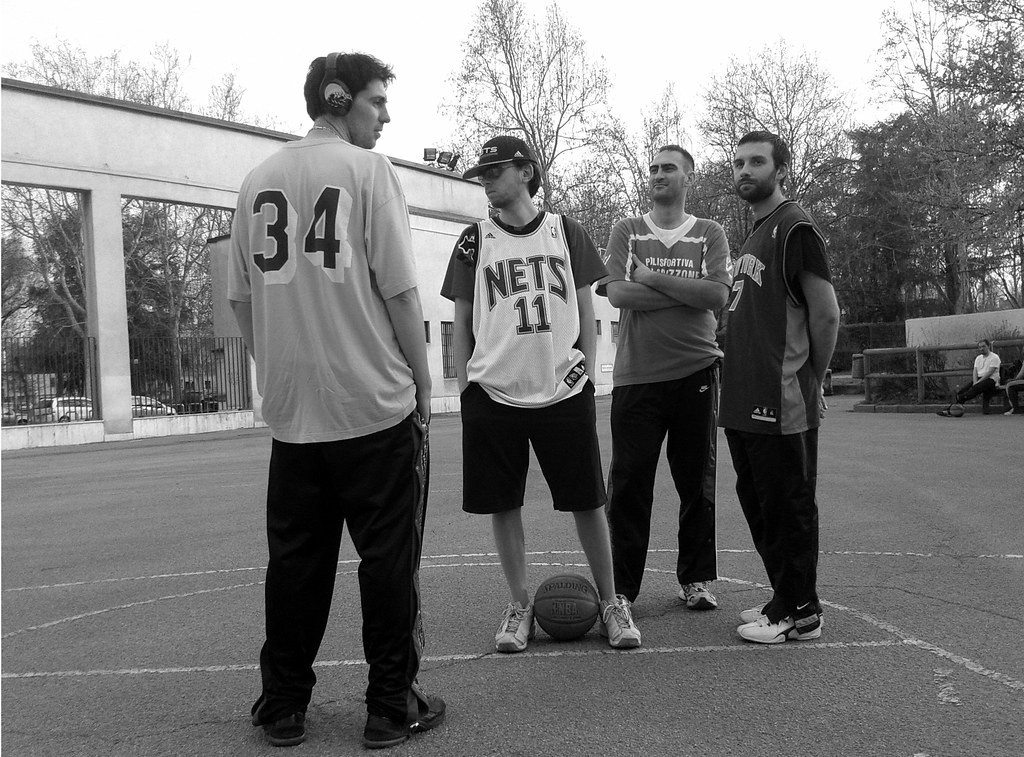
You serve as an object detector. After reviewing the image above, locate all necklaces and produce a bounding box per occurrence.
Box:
[307,126,341,139]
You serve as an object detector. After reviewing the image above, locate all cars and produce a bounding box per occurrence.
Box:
[1,408,27,426]
[27,397,92,423]
[19,402,27,410]
[132,396,178,417]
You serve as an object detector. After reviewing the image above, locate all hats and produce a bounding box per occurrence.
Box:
[463,136,537,179]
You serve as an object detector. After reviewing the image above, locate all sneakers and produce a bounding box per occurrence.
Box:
[599,594,641,648]
[737,600,824,643]
[494,601,536,652]
[678,582,717,609]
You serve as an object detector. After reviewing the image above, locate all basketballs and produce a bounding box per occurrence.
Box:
[533,573,601,640]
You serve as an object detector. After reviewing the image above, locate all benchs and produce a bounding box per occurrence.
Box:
[983,363,1022,414]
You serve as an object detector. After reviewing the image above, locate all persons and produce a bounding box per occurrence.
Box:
[713,131,839,643]
[602,145,733,615]
[439,136,640,653]
[1002,347,1024,416]
[225,51,446,750]
[935,339,1001,418]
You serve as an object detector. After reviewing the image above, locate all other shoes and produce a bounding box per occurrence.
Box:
[950,389,958,404]
[363,696,447,749]
[1004,407,1024,416]
[262,712,306,745]
[936,409,950,416]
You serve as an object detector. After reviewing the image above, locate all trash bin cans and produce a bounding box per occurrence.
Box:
[852,354,864,379]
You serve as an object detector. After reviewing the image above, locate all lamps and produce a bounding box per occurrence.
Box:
[448,155,460,171]
[423,148,436,167]
[437,152,452,168]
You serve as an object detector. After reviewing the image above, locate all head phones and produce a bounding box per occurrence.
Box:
[318,52,354,117]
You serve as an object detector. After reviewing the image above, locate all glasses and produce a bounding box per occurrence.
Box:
[478,166,518,180]
